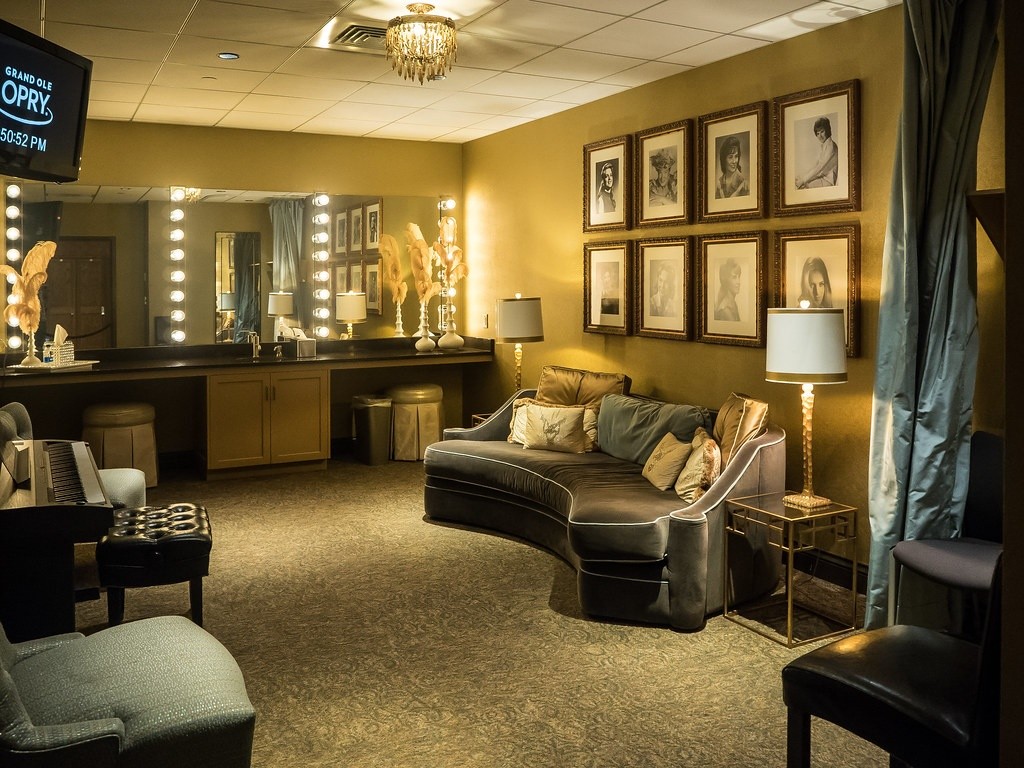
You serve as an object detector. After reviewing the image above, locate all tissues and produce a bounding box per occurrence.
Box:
[293,327,318,358]
[42,324,75,363]
[277,325,294,342]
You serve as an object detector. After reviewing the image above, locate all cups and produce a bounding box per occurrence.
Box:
[54,341,74,367]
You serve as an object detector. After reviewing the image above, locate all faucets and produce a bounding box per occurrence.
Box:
[250,332,257,342]
[253,335,262,358]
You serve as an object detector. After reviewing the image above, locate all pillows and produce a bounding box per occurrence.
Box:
[508,366,768,504]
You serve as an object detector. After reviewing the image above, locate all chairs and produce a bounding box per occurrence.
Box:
[0,613,255,768]
[781,431,1005,768]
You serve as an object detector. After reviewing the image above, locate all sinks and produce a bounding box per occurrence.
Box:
[234,356,290,362]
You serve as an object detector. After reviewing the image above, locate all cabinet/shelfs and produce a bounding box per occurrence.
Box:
[200,370,331,481]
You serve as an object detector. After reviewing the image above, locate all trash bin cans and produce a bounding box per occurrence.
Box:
[350,394,392,466]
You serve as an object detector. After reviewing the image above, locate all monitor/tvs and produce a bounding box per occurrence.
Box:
[0,19,93,184]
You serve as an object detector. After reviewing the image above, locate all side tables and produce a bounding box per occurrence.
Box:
[724,490,859,649]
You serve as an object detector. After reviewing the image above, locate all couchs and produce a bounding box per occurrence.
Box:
[424,388,786,631]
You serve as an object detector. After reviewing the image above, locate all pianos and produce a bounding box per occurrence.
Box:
[0,439,113,645]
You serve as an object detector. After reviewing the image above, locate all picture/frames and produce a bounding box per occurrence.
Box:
[583,80,863,360]
[332,198,383,317]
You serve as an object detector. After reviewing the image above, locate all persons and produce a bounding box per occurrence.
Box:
[716,137,748,197]
[714,258,742,321]
[796,117,838,189]
[597,163,616,213]
[798,258,834,307]
[600,267,612,296]
[649,150,677,206]
[650,260,676,316]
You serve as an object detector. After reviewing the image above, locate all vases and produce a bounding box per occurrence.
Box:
[393,304,406,337]
[21,332,42,367]
[415,297,465,353]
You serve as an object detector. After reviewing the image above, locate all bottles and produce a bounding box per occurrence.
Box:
[43,345,53,363]
[43,338,53,350]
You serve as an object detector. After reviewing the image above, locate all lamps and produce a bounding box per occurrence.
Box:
[267,291,295,335]
[766,300,849,514]
[385,3,458,85]
[498,294,544,393]
[335,291,368,338]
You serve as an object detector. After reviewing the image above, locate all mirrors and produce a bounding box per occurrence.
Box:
[6,179,440,357]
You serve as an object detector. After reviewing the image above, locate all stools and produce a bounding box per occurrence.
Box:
[96,502,212,626]
[82,403,159,488]
[390,382,444,461]
